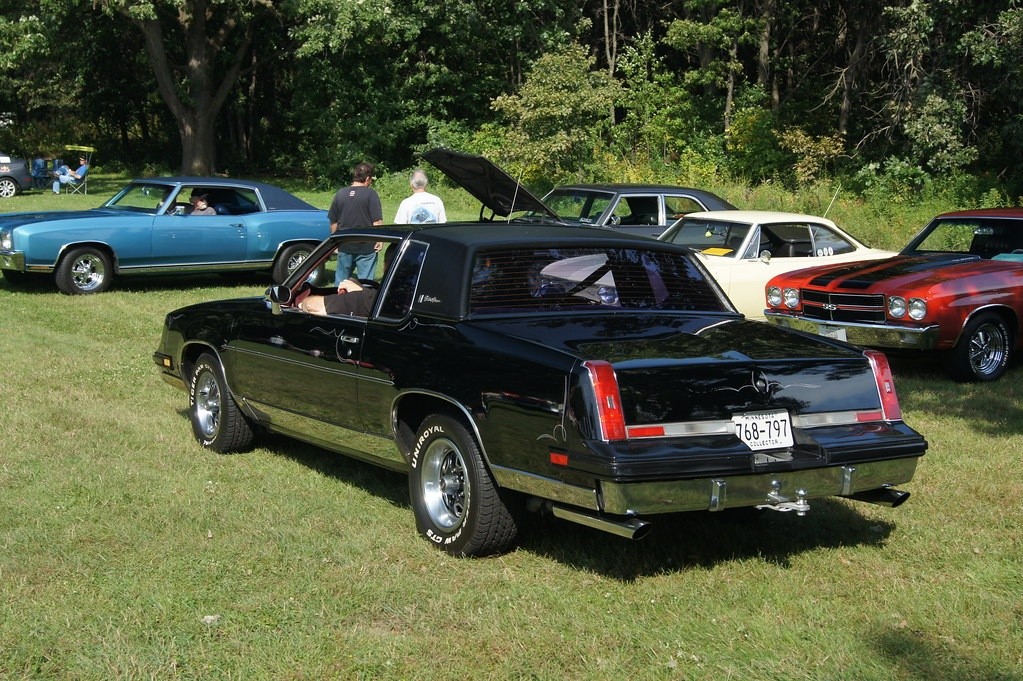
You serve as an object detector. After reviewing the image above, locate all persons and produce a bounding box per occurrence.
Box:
[31,155,49,188]
[155,191,177,215]
[394,170,447,223]
[51,155,89,195]
[190,189,216,215]
[299,243,399,316]
[327,163,383,287]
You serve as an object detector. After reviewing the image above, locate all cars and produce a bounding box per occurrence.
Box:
[764,208,1023,390]
[152,220,932,558]
[419,148,742,243]
[535,211,898,323]
[0,151,32,199]
[0,177,340,296]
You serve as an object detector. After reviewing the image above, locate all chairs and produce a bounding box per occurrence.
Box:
[772,243,794,257]
[215,205,229,215]
[637,213,657,225]
[31,158,90,196]
[982,224,1011,256]
